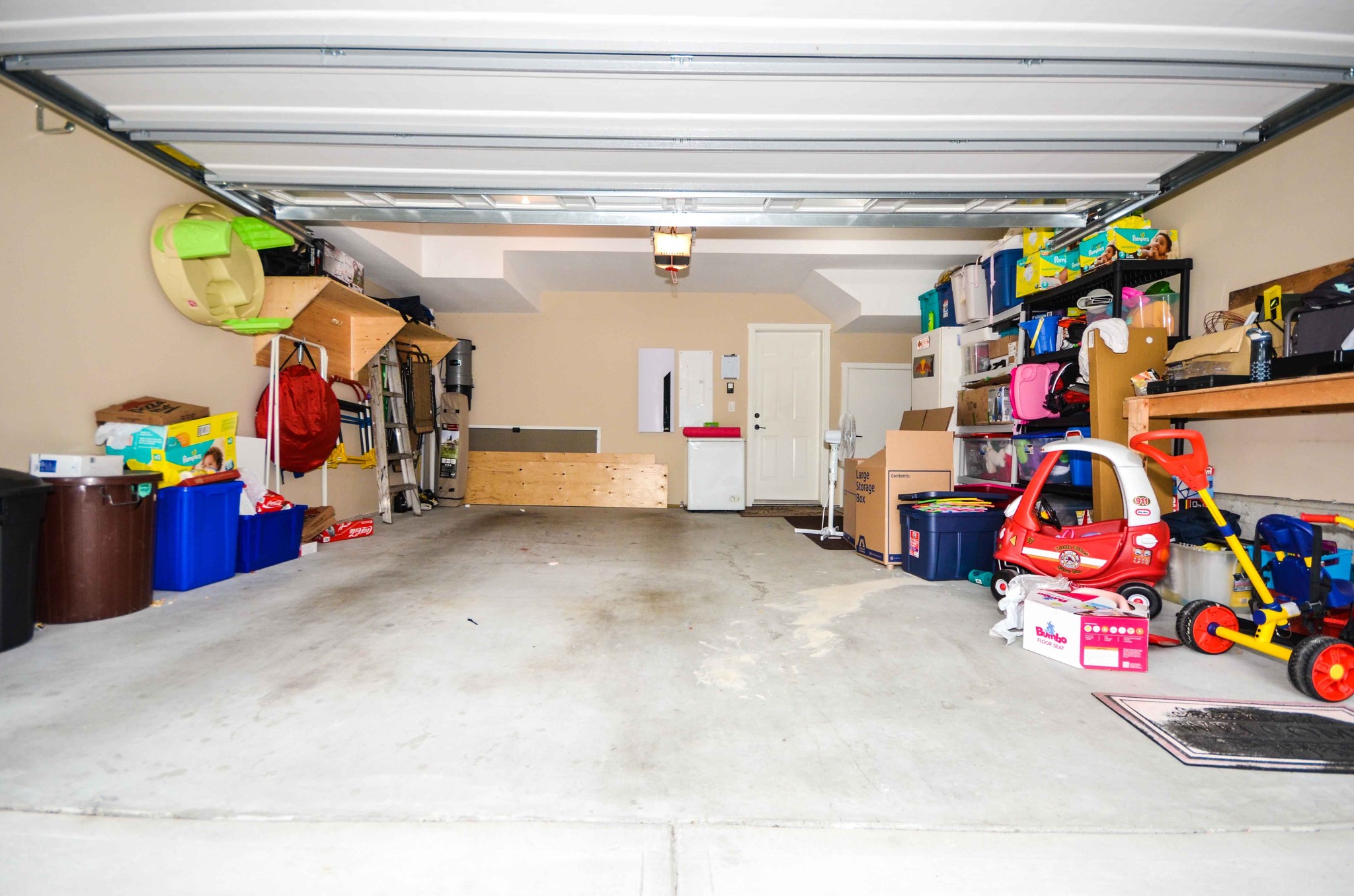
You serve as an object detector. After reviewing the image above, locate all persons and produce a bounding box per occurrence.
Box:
[1094,244,1116,271]
[1066,240,1080,252]
[1044,266,1068,285]
[200,446,224,472]
[1138,232,1172,260]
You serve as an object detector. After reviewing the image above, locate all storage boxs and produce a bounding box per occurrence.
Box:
[235,503,308,573]
[843,457,870,549]
[1151,541,1253,615]
[1246,545,1354,590]
[255,488,284,514]
[897,491,1012,582]
[298,506,374,557]
[28,452,125,478]
[955,432,1020,483]
[0,465,56,656]
[305,238,365,294]
[1163,289,1297,377]
[1063,426,1093,486]
[152,481,244,593]
[104,407,240,501]
[987,384,1014,426]
[1011,430,1072,484]
[35,469,165,624]
[956,385,990,427]
[95,395,210,426]
[917,198,1181,376]
[1022,588,1150,674]
[853,430,955,570]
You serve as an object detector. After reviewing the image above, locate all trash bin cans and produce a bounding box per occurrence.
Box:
[0,468,56,653]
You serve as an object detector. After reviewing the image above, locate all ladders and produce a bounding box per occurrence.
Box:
[365,337,423,525]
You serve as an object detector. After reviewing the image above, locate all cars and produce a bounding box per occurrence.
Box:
[991,430,1170,619]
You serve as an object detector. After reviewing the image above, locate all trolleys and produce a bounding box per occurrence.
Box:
[1128,429,1353,703]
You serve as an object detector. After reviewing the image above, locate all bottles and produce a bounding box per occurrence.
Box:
[1245,326,1275,383]
[967,568,992,586]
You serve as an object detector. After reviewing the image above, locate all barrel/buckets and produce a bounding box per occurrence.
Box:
[26,465,164,626]
[1018,313,1062,355]
[1,463,54,655]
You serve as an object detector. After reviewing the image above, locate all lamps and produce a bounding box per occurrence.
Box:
[650,226,697,284]
